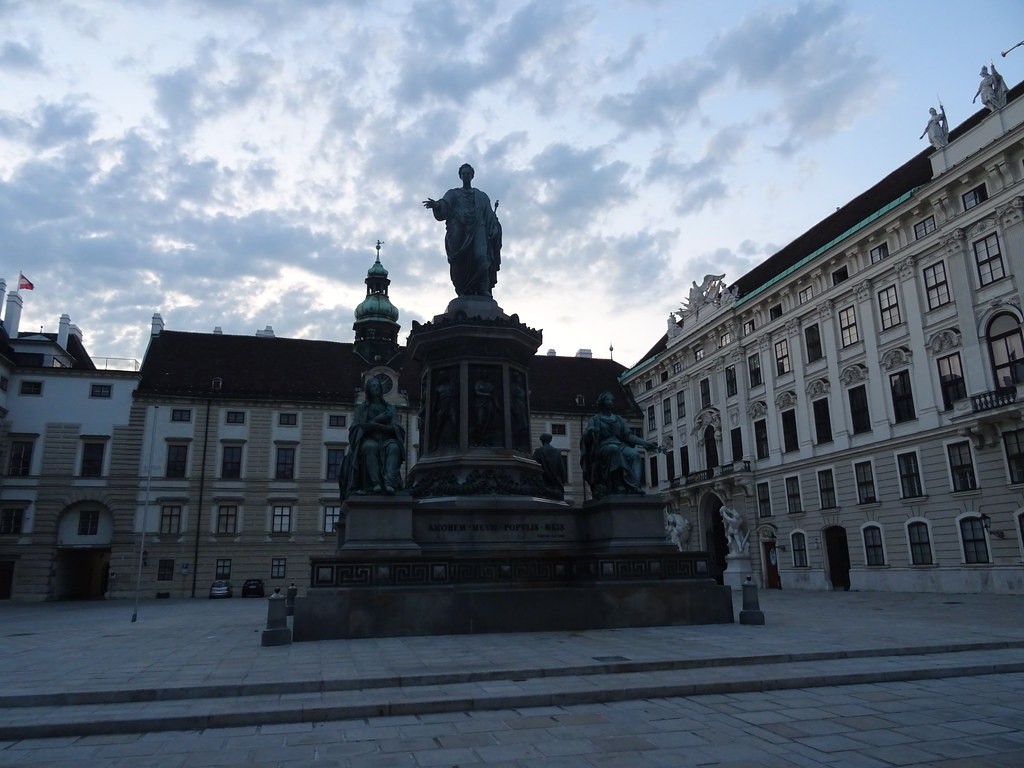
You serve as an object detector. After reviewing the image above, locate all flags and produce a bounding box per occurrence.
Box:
[18,272,34,290]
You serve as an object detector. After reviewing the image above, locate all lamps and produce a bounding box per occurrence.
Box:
[769,532,785,552]
[110,569,116,581]
[25,510,31,519]
[813,538,820,550]
[979,513,1004,539]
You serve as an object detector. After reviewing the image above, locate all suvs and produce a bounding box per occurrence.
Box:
[242,578,265,599]
[209,579,234,599]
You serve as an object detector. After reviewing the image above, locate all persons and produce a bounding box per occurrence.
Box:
[971,65,1001,114]
[723,508,744,552]
[579,393,659,500]
[422,162,503,298]
[919,105,949,150]
[533,432,567,501]
[431,370,458,452]
[475,368,503,449]
[418,381,427,457]
[669,312,676,320]
[349,379,406,495]
[720,282,731,295]
[664,523,682,551]
[509,370,527,448]
